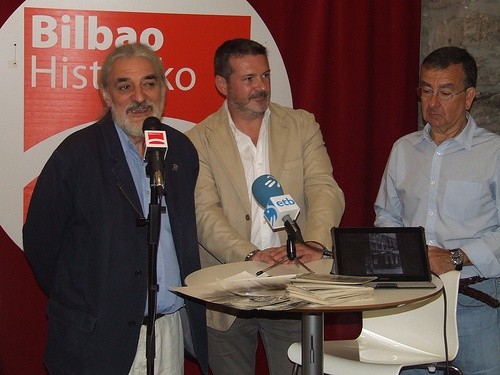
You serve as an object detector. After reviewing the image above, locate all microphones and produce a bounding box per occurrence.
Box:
[142,116,169,196]
[252,174,304,243]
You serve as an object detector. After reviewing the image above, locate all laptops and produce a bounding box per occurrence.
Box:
[331,226,437,289]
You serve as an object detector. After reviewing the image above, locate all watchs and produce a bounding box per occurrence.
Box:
[449,248,464,271]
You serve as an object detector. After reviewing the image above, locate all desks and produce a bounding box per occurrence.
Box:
[185,259,443,375]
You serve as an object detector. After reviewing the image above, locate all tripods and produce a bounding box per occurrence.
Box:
[256,220,316,276]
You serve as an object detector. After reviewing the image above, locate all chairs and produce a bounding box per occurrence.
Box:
[288,270,462,375]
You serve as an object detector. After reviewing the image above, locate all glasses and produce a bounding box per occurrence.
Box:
[415,85,469,99]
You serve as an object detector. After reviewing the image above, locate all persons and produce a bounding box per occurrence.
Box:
[183,38,346,375]
[374,46,500,375]
[23,43,210,374]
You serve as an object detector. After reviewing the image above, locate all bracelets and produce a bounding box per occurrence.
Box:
[245,249,261,261]
